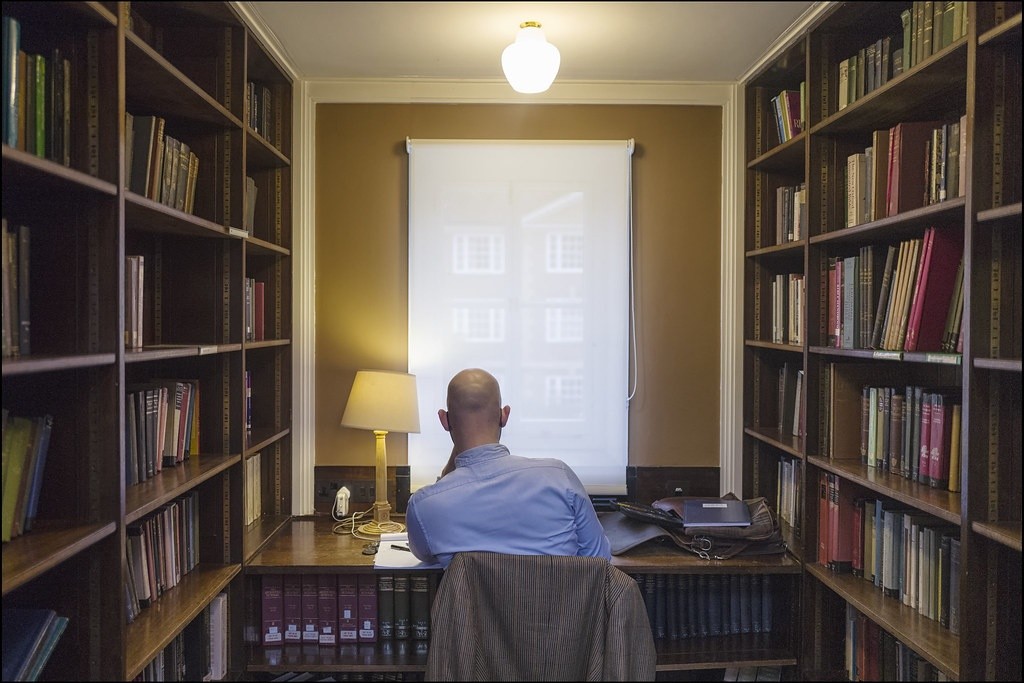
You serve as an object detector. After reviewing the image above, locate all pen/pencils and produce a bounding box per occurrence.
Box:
[390,544,411,553]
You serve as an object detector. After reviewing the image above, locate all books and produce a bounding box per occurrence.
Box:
[627,0,1024,683]
[0,0,437,683]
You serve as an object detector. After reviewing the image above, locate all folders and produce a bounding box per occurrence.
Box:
[682,498,753,527]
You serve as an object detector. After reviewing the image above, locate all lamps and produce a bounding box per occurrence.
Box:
[340,371,421,536]
[501,22,561,94]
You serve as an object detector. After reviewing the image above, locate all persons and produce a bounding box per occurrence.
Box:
[406,368,612,571]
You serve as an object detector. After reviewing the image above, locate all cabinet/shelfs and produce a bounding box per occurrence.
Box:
[243,513,803,682]
[741,1,1022,681]
[0,1,294,683]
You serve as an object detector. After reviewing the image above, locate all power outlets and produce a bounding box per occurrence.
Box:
[339,479,392,504]
